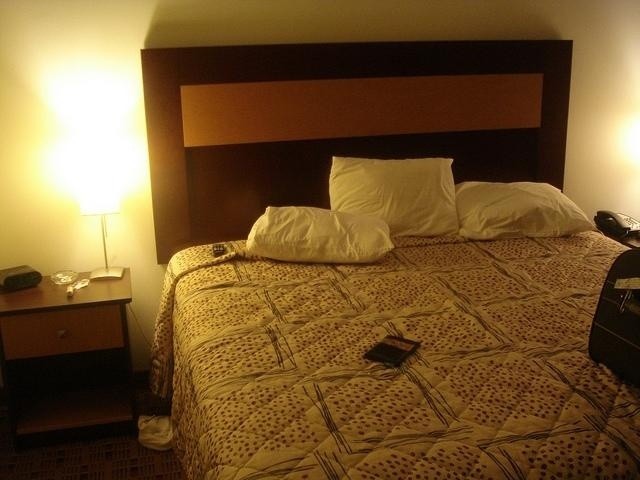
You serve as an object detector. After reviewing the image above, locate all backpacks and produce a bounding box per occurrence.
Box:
[586,246,640,390]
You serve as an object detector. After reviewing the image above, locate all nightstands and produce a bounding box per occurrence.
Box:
[0,267,142,439]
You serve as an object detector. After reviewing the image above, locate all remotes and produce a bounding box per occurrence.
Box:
[213,243,224,255]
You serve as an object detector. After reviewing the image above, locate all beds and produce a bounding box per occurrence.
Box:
[141,38,638,478]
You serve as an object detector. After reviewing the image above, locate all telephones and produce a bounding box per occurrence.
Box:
[594,210,640,240]
